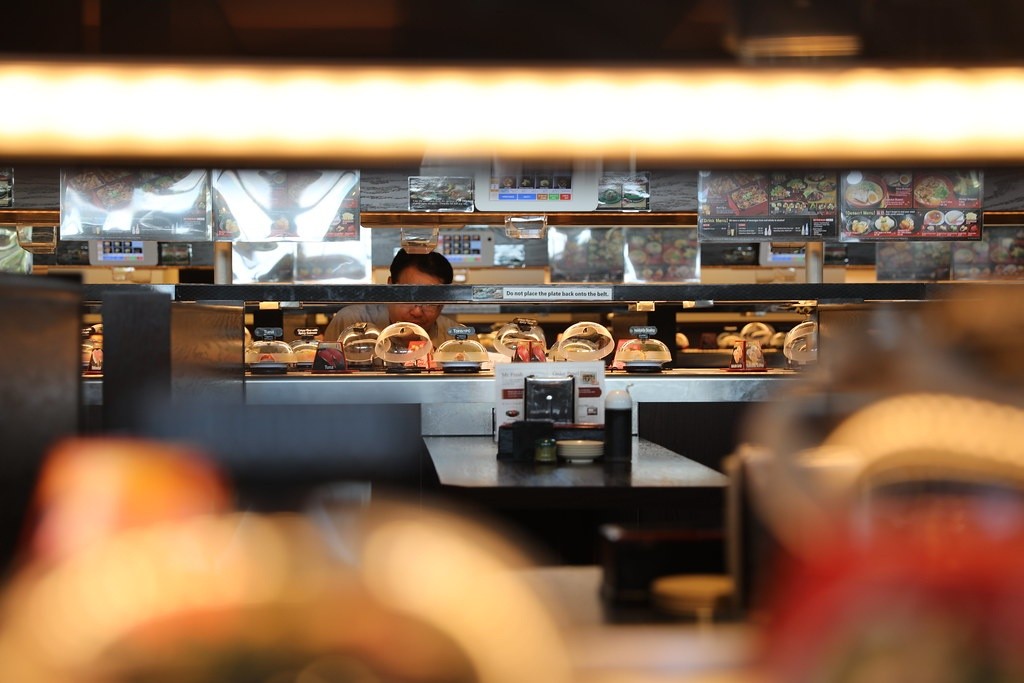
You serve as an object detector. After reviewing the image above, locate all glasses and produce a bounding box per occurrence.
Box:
[397,303,443,311]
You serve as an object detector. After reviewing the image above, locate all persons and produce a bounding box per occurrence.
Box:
[328,249,480,366]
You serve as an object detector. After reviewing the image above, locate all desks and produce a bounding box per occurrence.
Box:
[421,434,732,623]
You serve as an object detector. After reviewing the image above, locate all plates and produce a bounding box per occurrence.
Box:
[556,440,603,463]
[871,215,897,234]
[945,211,964,227]
[846,217,872,235]
[770,172,836,201]
[898,214,921,234]
[924,211,944,225]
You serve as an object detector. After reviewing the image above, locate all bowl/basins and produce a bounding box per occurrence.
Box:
[914,174,954,207]
[845,176,885,207]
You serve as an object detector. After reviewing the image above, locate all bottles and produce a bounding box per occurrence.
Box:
[602,390,633,466]
[534,439,558,465]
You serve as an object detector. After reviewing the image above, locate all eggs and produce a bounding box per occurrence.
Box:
[867,191,878,204]
[899,174,909,184]
